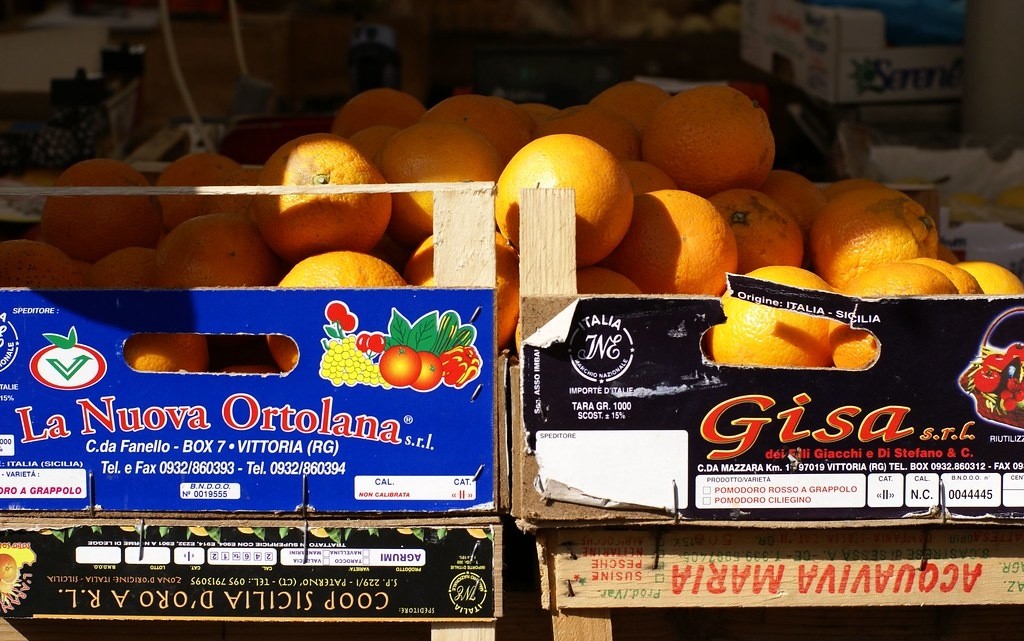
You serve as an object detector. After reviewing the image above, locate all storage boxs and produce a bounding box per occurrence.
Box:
[0,177,507,516]
[521,189,1024,529]
[737,0,974,108]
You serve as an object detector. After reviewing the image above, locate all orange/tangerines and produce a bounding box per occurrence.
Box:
[0,81,1023,373]
